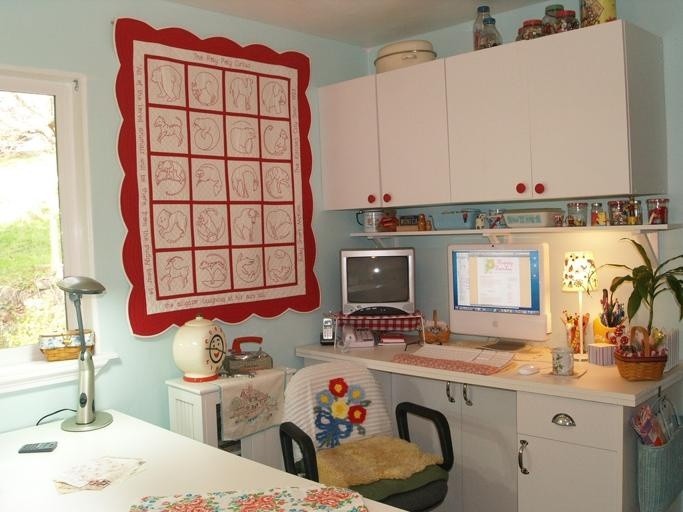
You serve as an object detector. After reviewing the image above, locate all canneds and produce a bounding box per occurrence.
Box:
[607,200,642,225]
[567,202,588,226]
[647,198,669,226]
[554,215,562,227]
[591,204,602,225]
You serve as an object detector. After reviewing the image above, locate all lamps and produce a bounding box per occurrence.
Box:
[55,274,114,434]
[559,249,603,382]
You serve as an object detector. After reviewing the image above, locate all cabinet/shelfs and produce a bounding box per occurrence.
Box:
[314,56,450,213]
[165,363,296,472]
[443,17,667,208]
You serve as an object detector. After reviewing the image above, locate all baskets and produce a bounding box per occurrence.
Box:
[613,349,676,382]
[37,328,96,363]
[636,397,683,512]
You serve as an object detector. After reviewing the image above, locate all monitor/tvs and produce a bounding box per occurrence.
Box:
[340,248,416,316]
[447,242,552,349]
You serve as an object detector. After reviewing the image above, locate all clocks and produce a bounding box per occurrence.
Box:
[171,313,226,383]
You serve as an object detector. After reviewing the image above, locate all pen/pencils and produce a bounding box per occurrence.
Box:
[600,290,628,328]
[561,305,590,353]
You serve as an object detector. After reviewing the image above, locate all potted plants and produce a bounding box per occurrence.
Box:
[586,232,683,383]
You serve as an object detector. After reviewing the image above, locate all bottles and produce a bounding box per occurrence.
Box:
[417,214,432,231]
[514,4,578,42]
[473,4,502,49]
[565,197,670,227]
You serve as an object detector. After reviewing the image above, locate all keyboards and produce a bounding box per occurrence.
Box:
[409,343,515,368]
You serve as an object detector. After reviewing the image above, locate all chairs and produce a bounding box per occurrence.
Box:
[277,359,454,511]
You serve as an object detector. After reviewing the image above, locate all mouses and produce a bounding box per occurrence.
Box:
[519,363,540,375]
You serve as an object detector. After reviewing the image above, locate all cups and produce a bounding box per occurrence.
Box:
[355,208,383,232]
[550,345,574,376]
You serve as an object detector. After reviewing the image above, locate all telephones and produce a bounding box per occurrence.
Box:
[342,325,374,348]
[320,317,335,345]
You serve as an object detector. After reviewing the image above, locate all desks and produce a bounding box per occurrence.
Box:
[295,330,683,512]
[0,407,406,511]
